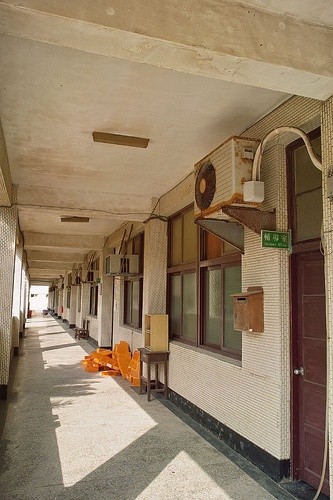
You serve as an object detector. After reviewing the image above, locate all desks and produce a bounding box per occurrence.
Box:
[137,348,171,401]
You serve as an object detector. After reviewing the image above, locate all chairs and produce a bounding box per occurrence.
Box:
[74,319,86,339]
[77,320,90,340]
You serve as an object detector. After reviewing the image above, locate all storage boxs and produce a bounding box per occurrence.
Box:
[81,340,142,387]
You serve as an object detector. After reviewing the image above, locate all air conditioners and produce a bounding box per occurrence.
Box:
[192,135,265,224]
[104,254,140,281]
[49,269,101,292]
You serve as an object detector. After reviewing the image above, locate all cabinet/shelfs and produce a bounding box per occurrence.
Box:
[143,313,169,353]
